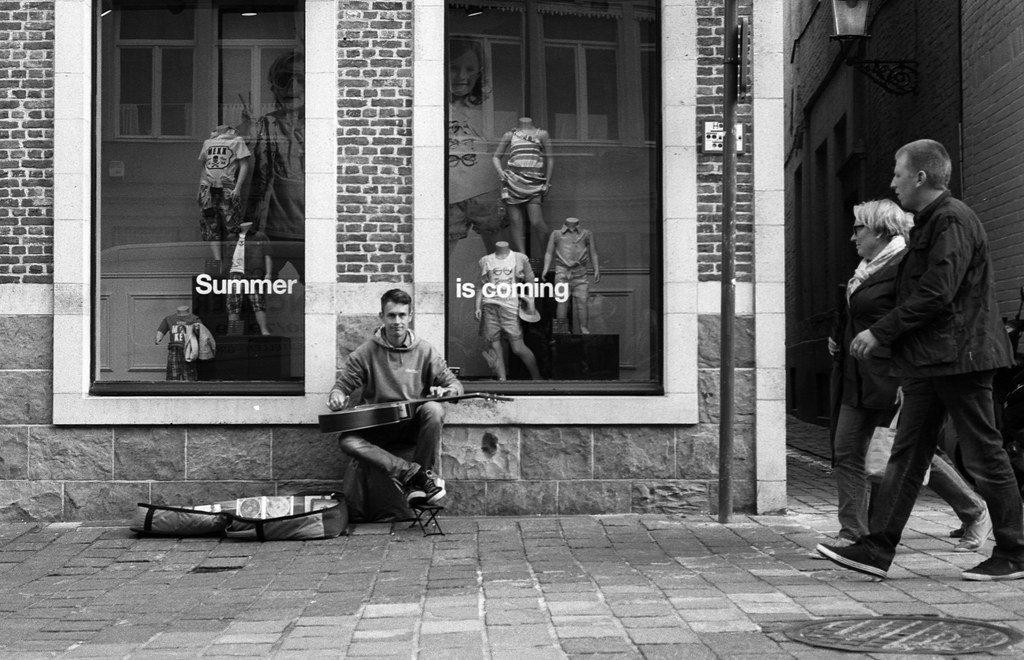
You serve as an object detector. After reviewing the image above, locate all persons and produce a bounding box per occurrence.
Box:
[817,139,1024,584]
[326,289,464,508]
[154,127,269,382]
[447,36,519,255]
[810,200,993,560]
[236,53,305,335]
[474,118,600,381]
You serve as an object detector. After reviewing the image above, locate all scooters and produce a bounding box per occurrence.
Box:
[954,284,1024,491]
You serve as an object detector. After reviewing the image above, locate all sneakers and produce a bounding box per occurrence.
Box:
[423,470,447,506]
[404,481,427,504]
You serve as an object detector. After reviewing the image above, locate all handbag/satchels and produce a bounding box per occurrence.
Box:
[864,406,933,487]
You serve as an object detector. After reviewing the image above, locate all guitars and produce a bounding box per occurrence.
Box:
[318,392,514,435]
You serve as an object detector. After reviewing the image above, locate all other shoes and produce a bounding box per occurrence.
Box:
[961,555,1024,581]
[954,507,993,552]
[816,534,892,579]
[808,536,855,560]
[950,528,965,537]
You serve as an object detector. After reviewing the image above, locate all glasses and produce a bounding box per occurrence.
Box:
[853,224,865,236]
